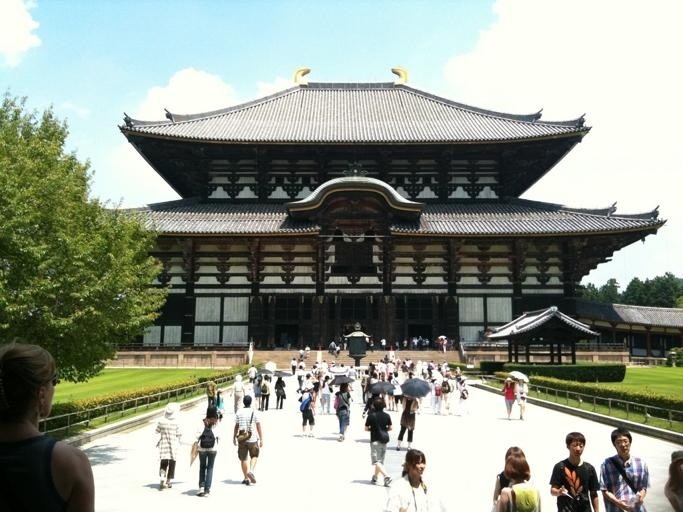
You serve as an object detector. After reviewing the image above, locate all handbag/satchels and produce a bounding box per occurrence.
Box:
[372,413,390,445]
[237,412,255,441]
[332,393,340,409]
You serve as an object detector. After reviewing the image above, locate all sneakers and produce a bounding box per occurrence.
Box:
[247,473,256,484]
[203,488,209,496]
[371,476,378,484]
[197,486,204,495]
[159,481,166,488]
[167,482,174,489]
[383,476,392,487]
[241,479,248,484]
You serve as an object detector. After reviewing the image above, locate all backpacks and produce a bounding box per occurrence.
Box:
[434,384,441,396]
[262,383,268,394]
[441,379,450,394]
[461,389,469,400]
[199,426,216,449]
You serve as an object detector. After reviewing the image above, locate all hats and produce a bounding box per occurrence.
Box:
[504,378,513,384]
[162,402,180,421]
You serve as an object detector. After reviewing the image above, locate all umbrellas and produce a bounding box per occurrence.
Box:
[257,369,273,375]
[328,376,355,386]
[368,382,396,395]
[189,438,199,467]
[273,371,292,378]
[509,371,530,383]
[438,335,447,340]
[401,378,432,399]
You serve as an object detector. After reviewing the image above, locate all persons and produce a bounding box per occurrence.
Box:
[383,449,432,512]
[490,447,541,512]
[302,373,317,416]
[370,333,457,354]
[154,403,182,489]
[275,378,286,409]
[365,399,393,488]
[549,431,599,512]
[333,383,354,442]
[231,374,245,414]
[501,377,516,420]
[290,357,298,376]
[196,406,222,497]
[514,379,529,420]
[233,395,263,485]
[361,354,470,416]
[361,392,387,418]
[396,393,419,452]
[297,341,358,391]
[0,343,95,512]
[664,450,683,512]
[261,375,271,411]
[206,377,217,408]
[599,429,650,511]
[253,378,262,410]
[299,382,316,437]
[320,376,333,416]
